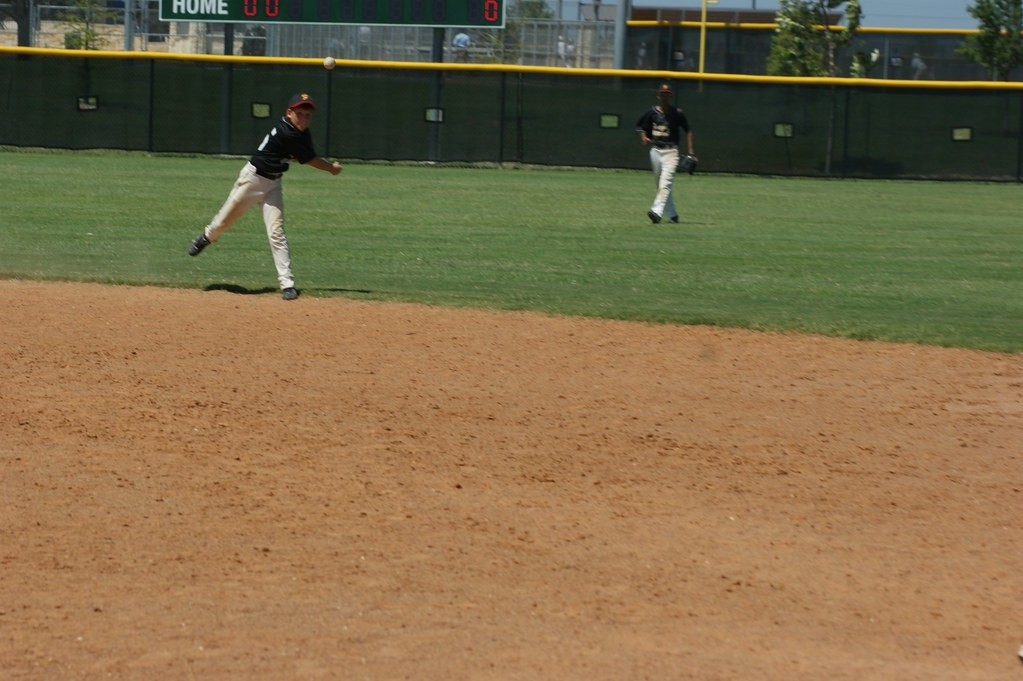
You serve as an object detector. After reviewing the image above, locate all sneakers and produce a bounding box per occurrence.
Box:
[647,210,661,223]
[188,232,211,256]
[670,216,679,223]
[283,287,297,300]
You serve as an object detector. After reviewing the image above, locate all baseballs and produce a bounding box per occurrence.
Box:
[323,56,336,70]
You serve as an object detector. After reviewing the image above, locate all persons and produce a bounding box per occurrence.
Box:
[452,30,470,63]
[910,51,934,80]
[186,94,344,300]
[633,84,698,225]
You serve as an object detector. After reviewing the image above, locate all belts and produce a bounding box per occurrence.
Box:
[256,170,283,180]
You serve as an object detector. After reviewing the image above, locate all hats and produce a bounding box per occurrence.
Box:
[288,92,317,109]
[656,83,672,94]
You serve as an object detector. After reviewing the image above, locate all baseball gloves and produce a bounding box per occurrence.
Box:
[676,151,699,175]
